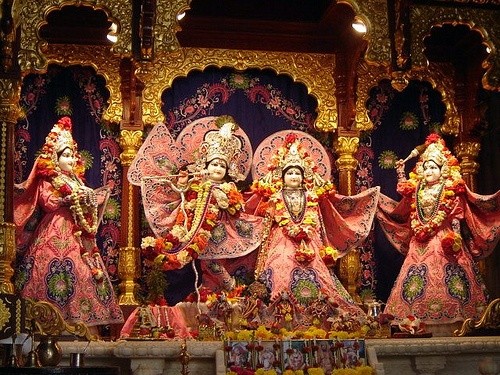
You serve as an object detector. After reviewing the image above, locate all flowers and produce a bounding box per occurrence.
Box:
[250,133,337,262]
[36,116,99,235]
[396,134,466,242]
[139,182,248,272]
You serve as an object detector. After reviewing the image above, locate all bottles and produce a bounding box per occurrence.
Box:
[35,334,62,367]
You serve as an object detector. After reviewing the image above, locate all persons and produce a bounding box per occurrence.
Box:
[377,134,499,335]
[169,121,265,294]
[15,119,125,339]
[251,133,380,328]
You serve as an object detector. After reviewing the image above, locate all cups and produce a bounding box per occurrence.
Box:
[69,353,84,369]
